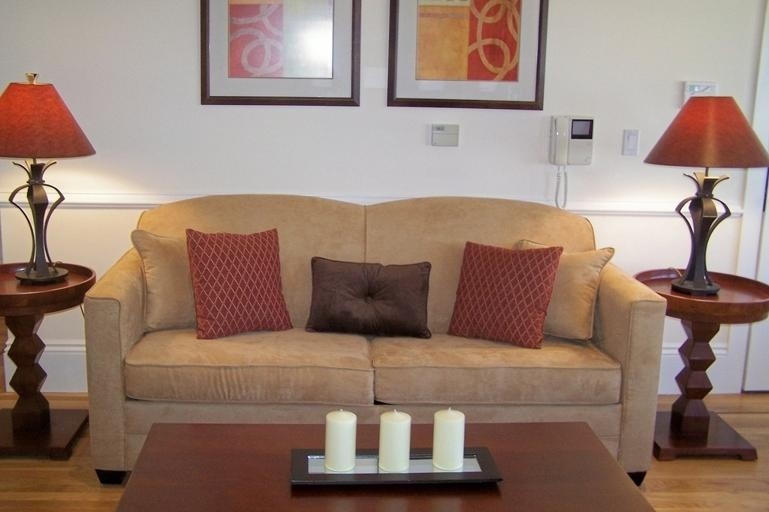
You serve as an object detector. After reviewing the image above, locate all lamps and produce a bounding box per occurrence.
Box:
[1,73,96,285]
[644,96,769,296]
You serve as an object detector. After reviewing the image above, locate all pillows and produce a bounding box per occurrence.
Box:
[446,241,564,349]
[305,257,432,339]
[185,229,294,340]
[130,230,196,333]
[521,240,615,345]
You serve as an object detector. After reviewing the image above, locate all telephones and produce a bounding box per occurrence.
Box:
[549,113,594,167]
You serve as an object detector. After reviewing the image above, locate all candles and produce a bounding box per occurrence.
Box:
[324,409,357,471]
[379,408,412,469]
[431,407,465,470]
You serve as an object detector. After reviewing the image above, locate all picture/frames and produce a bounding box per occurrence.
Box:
[200,1,362,106]
[387,0,549,110]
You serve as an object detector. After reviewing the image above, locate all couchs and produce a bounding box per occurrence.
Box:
[83,193,668,484]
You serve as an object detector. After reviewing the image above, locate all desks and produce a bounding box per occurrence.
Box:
[634,270,769,462]
[0,262,96,461]
